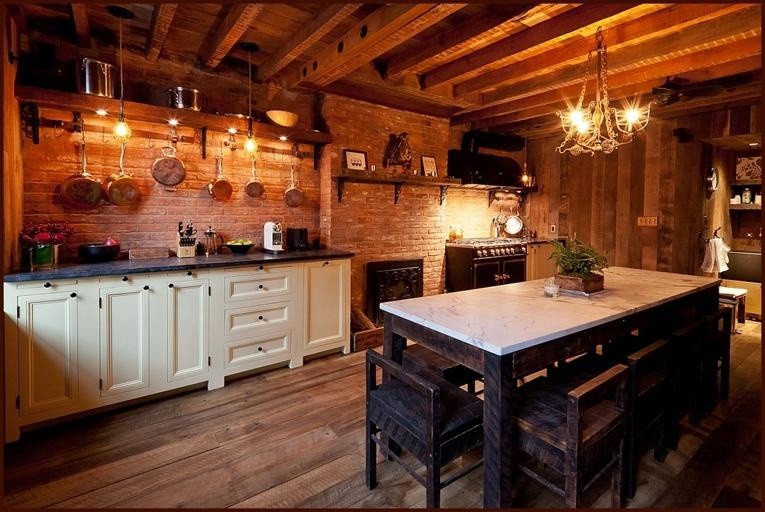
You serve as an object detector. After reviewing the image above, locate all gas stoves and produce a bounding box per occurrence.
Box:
[446,238,528,256]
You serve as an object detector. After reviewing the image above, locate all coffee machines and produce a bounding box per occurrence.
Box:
[261,221,287,255]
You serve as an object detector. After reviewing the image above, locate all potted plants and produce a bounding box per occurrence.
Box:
[546,230,607,294]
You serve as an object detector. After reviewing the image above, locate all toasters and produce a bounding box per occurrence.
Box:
[288,228,309,252]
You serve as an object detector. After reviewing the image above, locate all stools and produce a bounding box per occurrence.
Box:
[719,286,749,333]
[403,335,486,403]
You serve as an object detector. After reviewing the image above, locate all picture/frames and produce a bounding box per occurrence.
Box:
[341,148,370,172]
[420,154,439,178]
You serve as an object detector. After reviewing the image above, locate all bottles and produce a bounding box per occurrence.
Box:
[741,188,750,204]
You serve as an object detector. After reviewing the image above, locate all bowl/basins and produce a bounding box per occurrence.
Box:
[76,243,120,263]
[221,243,257,255]
[264,109,300,128]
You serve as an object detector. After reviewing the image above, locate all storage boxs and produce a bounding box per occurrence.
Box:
[128,245,170,260]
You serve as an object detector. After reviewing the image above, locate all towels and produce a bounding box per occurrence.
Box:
[699,236,731,274]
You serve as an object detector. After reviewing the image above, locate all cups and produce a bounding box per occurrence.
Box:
[313,240,321,251]
[544,279,561,298]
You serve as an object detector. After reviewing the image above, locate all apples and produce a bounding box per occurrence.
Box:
[226,239,252,245]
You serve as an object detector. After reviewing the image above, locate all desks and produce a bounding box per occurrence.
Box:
[376,265,723,509]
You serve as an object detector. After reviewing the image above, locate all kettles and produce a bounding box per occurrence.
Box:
[203,225,224,257]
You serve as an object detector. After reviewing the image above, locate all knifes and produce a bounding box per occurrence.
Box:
[178,220,195,246]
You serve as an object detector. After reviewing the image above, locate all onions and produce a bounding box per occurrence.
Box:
[102,237,117,245]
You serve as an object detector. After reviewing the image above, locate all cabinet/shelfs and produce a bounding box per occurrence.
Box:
[3,276,102,447]
[526,242,560,281]
[208,261,304,391]
[302,257,353,368]
[98,267,208,408]
[444,241,528,291]
[726,137,764,280]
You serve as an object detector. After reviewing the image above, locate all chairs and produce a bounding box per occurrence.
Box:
[566,305,732,483]
[508,364,633,510]
[358,339,489,508]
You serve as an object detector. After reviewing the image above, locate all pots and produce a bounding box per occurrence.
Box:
[495,203,524,235]
[245,154,264,198]
[207,154,235,203]
[60,138,105,211]
[164,82,203,111]
[152,145,187,193]
[282,161,304,207]
[100,142,139,206]
[78,55,118,98]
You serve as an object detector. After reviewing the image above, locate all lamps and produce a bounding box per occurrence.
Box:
[551,24,651,156]
[521,120,531,183]
[101,5,135,144]
[243,39,260,158]
